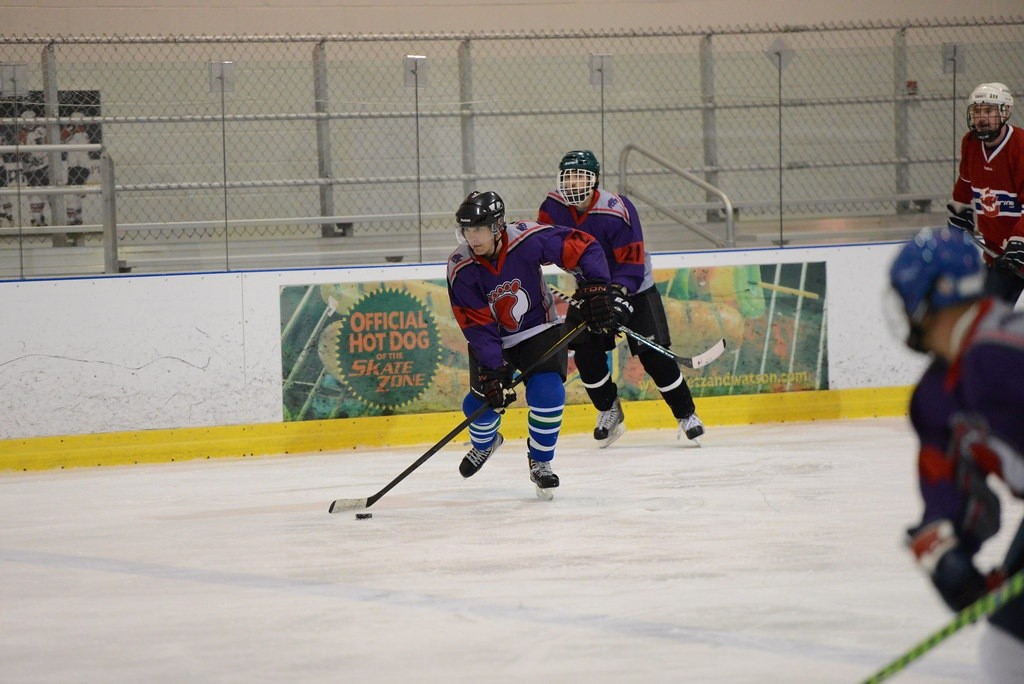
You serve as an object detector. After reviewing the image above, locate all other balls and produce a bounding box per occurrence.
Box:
[355,513,373,520]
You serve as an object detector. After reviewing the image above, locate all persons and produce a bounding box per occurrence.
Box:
[536,151,706,444]
[18,110,91,240]
[948,82,1023,283]
[445,191,611,501]
[888,229,1023,684]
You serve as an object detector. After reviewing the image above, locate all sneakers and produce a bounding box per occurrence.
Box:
[527,451,559,501]
[593,395,624,448]
[679,412,705,448]
[459,432,504,481]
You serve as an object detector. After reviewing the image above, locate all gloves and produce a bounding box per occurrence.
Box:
[610,287,634,333]
[584,283,617,334]
[904,517,987,617]
[479,367,518,415]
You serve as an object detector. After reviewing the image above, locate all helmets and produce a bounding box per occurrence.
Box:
[555,150,600,205]
[881,222,989,311]
[455,190,506,229]
[966,83,1015,142]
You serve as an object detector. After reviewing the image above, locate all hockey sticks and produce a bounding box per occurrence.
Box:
[967,231,1024,312]
[328,320,587,514]
[545,280,727,368]
[859,570,1024,684]
